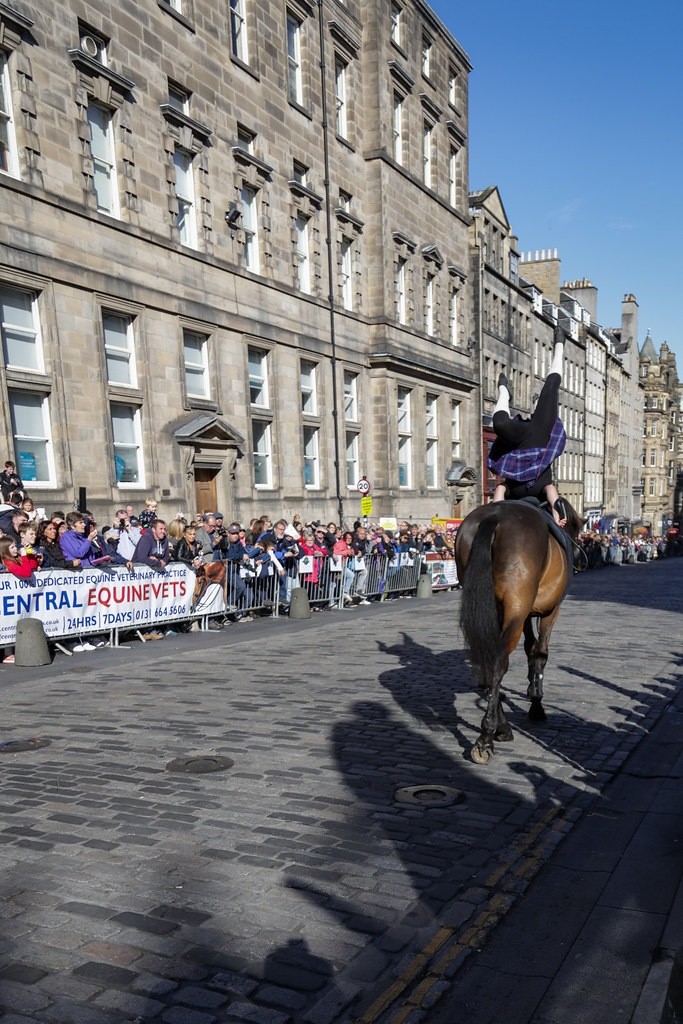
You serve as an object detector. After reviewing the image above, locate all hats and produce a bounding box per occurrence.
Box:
[228,523,240,534]
[316,526,328,533]
[212,512,223,519]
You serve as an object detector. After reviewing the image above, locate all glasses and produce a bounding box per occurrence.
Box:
[207,523,217,530]
[317,530,324,534]
[404,537,410,540]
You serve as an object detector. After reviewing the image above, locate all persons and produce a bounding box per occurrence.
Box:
[0,494,459,665]
[573,519,669,581]
[485,326,568,528]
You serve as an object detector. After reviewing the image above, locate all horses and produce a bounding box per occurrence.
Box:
[454,496,589,764]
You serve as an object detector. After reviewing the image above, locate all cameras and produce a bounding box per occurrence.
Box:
[119,519,126,529]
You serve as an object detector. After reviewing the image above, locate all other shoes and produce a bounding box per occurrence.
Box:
[308,590,415,613]
[553,325,566,353]
[142,629,179,640]
[2,655,15,664]
[208,596,291,630]
[497,372,512,402]
[71,639,111,653]
